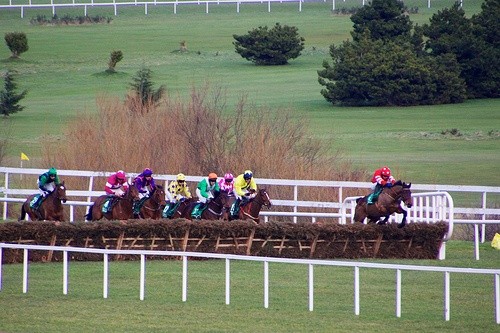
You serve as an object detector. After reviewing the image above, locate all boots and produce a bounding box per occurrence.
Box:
[367,193,375,205]
[231,200,237,215]
[31,195,43,207]
[135,199,143,212]
[162,204,170,217]
[191,203,201,218]
[101,200,110,212]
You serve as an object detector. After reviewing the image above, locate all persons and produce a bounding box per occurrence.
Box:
[230,169,258,220]
[32,166,60,209]
[101,170,130,213]
[190,172,220,220]
[130,168,156,215]
[218,172,234,195]
[161,173,192,217]
[371,166,396,202]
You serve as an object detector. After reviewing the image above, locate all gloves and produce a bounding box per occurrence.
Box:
[245,193,250,196]
[250,189,255,193]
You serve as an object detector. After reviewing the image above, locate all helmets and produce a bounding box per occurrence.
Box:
[116,170,126,182]
[224,173,233,184]
[48,167,58,178]
[381,166,390,180]
[176,173,185,182]
[209,172,217,181]
[143,168,152,177]
[244,170,253,181]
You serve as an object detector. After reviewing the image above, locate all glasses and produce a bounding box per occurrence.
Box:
[118,178,124,180]
[178,180,184,182]
[225,179,232,182]
[50,174,56,177]
[210,179,216,182]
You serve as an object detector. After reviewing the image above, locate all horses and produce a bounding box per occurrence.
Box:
[230,189,271,223]
[354,180,414,229]
[167,187,230,221]
[133,183,165,219]
[84,180,140,221]
[17,181,67,223]
